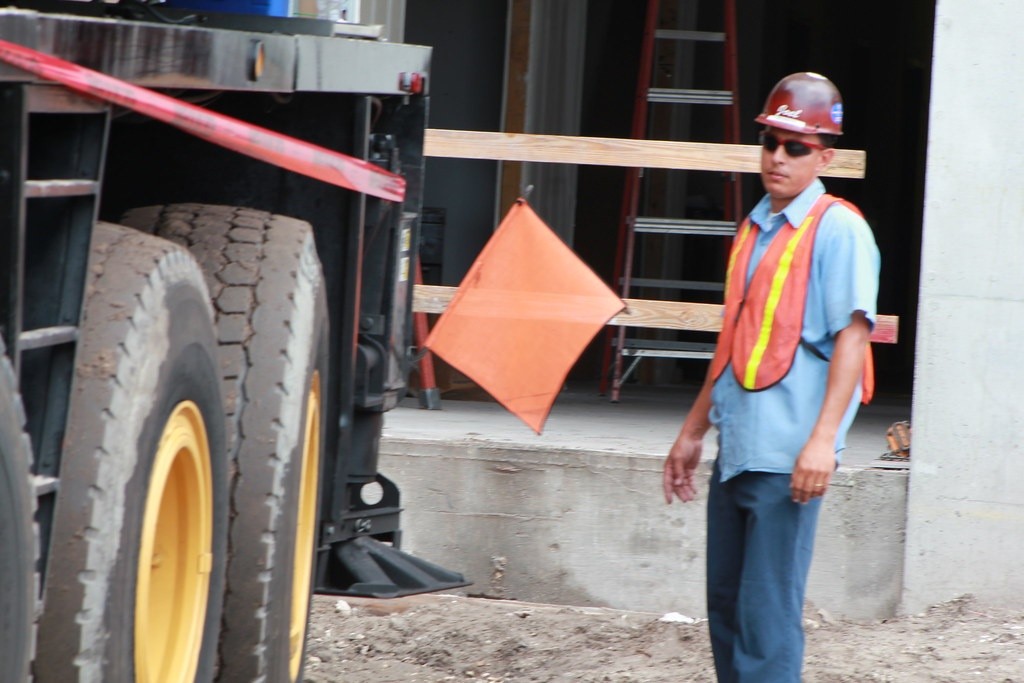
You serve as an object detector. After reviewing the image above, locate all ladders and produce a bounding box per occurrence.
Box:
[608,0,743,406]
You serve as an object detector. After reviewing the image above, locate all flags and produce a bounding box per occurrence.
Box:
[426,201,622,434]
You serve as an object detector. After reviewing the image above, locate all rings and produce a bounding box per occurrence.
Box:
[815,484,824,486]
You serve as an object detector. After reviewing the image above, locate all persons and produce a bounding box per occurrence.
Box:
[662,72,881,682]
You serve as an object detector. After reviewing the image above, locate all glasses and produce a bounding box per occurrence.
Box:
[757,131,833,156]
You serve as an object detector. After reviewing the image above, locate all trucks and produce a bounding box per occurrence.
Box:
[0,0,439,683]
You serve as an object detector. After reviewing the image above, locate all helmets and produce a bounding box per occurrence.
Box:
[753,71,845,136]
